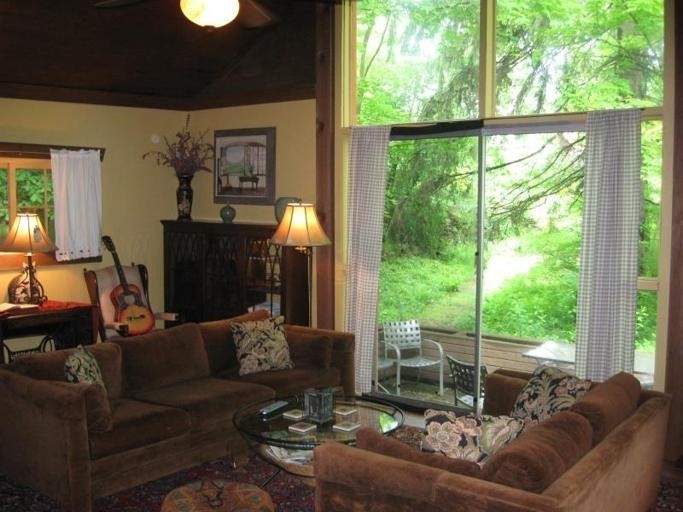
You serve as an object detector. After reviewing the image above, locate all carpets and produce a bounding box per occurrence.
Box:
[1,417,683,510]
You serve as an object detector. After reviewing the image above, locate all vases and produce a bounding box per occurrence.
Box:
[173,173,193,222]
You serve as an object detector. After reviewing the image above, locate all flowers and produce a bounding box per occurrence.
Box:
[138,113,218,176]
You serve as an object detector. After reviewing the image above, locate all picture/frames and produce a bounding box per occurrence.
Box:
[211,127,278,206]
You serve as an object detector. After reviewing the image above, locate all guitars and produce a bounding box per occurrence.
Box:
[103,235,156,335]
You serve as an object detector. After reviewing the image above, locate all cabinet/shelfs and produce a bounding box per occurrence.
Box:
[158,218,305,328]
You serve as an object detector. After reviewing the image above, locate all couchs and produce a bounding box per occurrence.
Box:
[310,367,670,510]
[1,306,353,511]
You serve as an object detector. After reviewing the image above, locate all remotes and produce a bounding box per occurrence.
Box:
[256,400,289,415]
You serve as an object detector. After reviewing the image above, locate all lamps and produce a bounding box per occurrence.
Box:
[177,0,240,37]
[0,209,52,306]
[275,203,327,326]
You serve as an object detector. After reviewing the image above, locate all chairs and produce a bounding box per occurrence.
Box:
[375,337,403,396]
[81,264,179,342]
[383,319,449,398]
[444,354,488,409]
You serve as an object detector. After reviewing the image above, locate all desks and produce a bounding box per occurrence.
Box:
[523,339,580,368]
[0,298,97,364]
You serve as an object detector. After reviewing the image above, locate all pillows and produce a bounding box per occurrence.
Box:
[567,371,644,443]
[479,411,593,492]
[61,341,104,386]
[358,422,484,474]
[232,316,296,376]
[415,406,515,462]
[510,363,594,429]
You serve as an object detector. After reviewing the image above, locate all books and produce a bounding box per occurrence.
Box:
[0,303,40,312]
[260,431,320,467]
